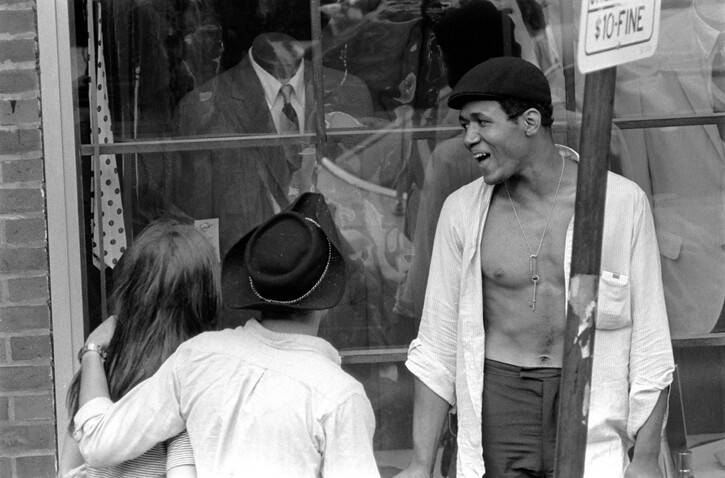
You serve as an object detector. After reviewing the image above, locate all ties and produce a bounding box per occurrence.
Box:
[276,85,301,173]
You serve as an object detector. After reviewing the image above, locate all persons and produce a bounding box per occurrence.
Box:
[610,0,725,335]
[166,37,375,322]
[393,0,635,447]
[392,57,675,478]
[73,193,383,478]
[65,213,224,478]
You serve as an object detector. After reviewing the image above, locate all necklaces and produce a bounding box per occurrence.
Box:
[505,149,566,310]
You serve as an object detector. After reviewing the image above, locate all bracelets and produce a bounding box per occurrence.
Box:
[76,342,107,363]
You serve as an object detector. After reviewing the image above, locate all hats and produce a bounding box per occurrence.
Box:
[447,56,550,105]
[219,191,347,310]
